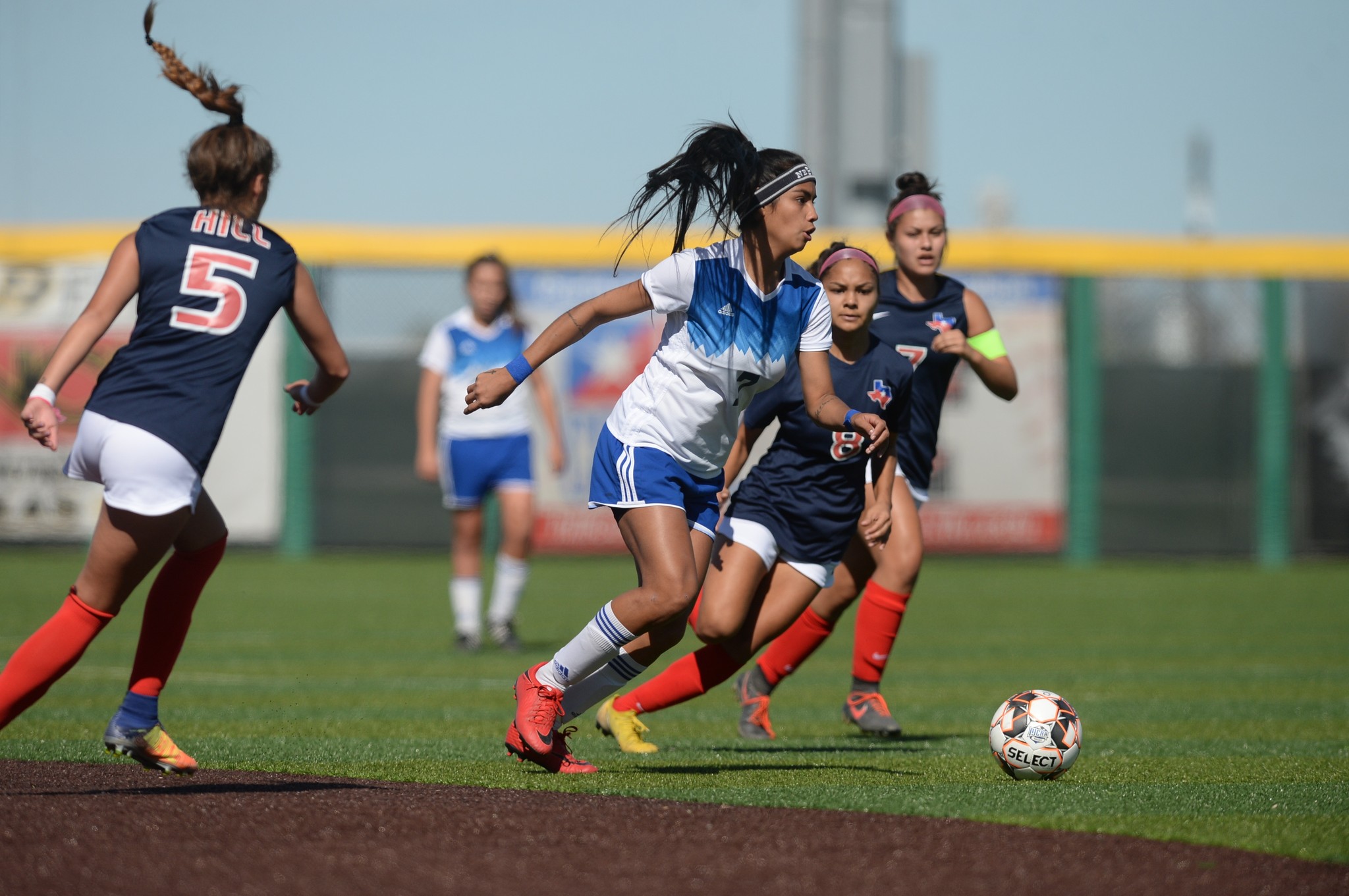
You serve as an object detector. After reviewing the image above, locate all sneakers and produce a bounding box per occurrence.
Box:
[840,691,900,736]
[733,671,776,740]
[510,661,565,755]
[504,718,600,774]
[103,705,198,777]
[492,623,518,651]
[596,694,657,754]
[458,634,483,655]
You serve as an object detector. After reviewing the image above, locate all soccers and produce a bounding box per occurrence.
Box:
[988,689,1081,779]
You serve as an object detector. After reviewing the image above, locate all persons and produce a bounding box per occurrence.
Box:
[1,0,347,777]
[463,109,894,776]
[416,254,569,654]
[731,172,1023,736]
[593,238,912,755]
